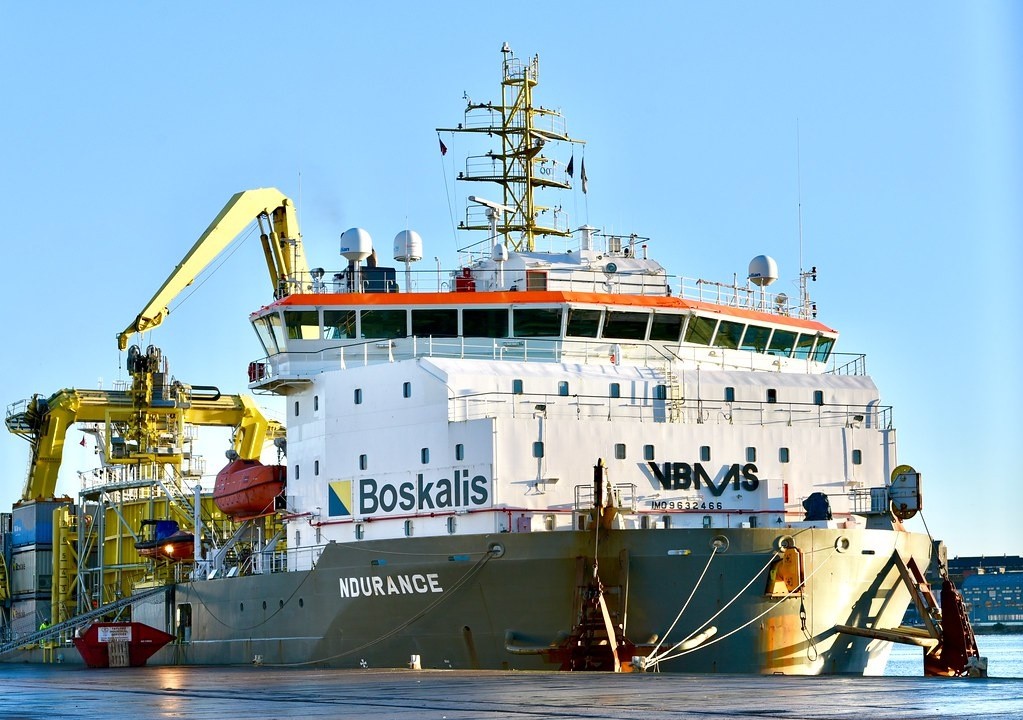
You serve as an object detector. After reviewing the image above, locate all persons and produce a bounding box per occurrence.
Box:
[40,618,52,646]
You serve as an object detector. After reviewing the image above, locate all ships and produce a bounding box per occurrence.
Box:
[0,41,936,676]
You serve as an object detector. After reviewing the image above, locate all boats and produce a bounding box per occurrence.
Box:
[134,517,199,562]
[213,455,287,523]
